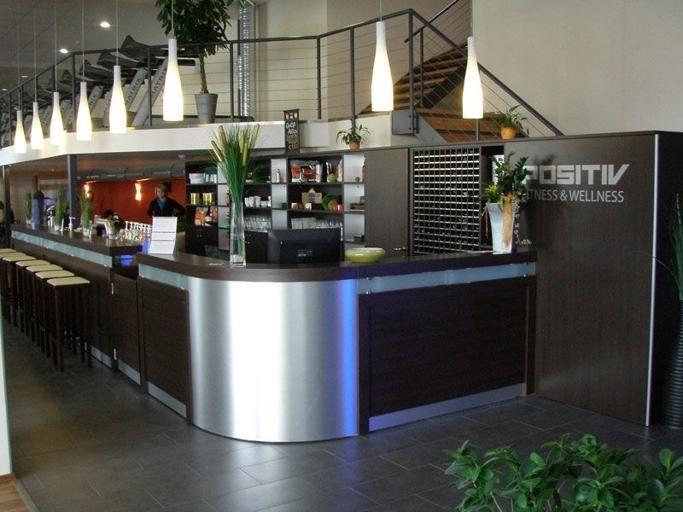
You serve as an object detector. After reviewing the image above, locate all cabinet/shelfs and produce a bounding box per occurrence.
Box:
[185,148,408,263]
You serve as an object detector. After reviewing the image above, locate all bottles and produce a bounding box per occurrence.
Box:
[336,161,343,181]
[205,206,213,226]
[276,169,280,182]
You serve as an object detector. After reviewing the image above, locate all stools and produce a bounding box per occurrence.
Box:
[26,265,63,342]
[16,260,50,331]
[0,249,16,295]
[0,252,25,318]
[35,271,75,356]
[47,277,90,373]
[3,255,36,327]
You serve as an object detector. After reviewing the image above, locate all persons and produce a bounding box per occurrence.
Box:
[105,211,126,230]
[0,201,7,248]
[147,184,186,217]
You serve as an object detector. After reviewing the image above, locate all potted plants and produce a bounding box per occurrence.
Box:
[212,125,260,268]
[495,105,528,139]
[80,198,92,237]
[485,152,528,255]
[53,192,66,232]
[23,193,32,226]
[157,0,235,124]
[337,125,369,149]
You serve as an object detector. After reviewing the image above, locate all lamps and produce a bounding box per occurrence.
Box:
[14,24,26,152]
[462,0,484,120]
[50,0,62,144]
[75,0,91,141]
[109,0,126,134]
[371,1,392,112]
[30,15,43,150]
[163,1,183,122]
